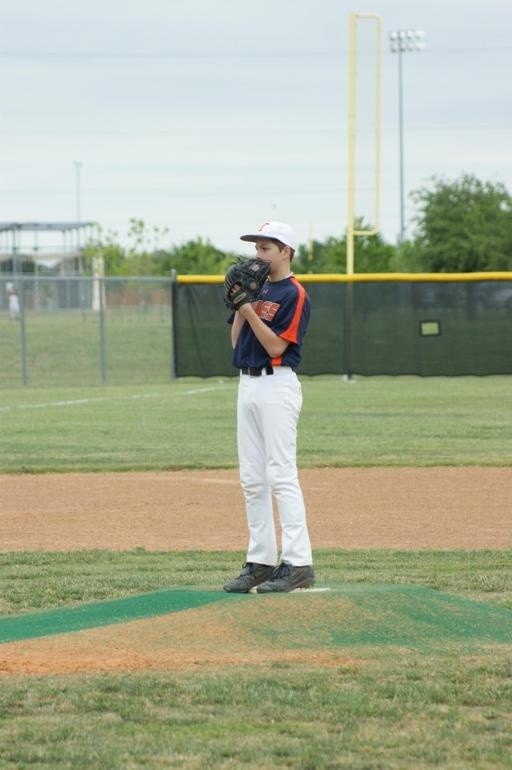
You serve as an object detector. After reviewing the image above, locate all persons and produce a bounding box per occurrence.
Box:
[5,281,21,322]
[223,221,316,594]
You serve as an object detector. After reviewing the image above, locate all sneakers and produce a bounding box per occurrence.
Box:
[247,563,316,593]
[222,562,277,593]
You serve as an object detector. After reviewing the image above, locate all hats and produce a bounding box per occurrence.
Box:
[238,219,299,252]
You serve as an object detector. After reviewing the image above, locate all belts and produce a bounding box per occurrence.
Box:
[240,365,274,377]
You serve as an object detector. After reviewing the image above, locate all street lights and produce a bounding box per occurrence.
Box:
[390,30,424,240]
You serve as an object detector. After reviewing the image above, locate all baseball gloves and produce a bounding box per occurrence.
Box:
[223,258,271,311]
